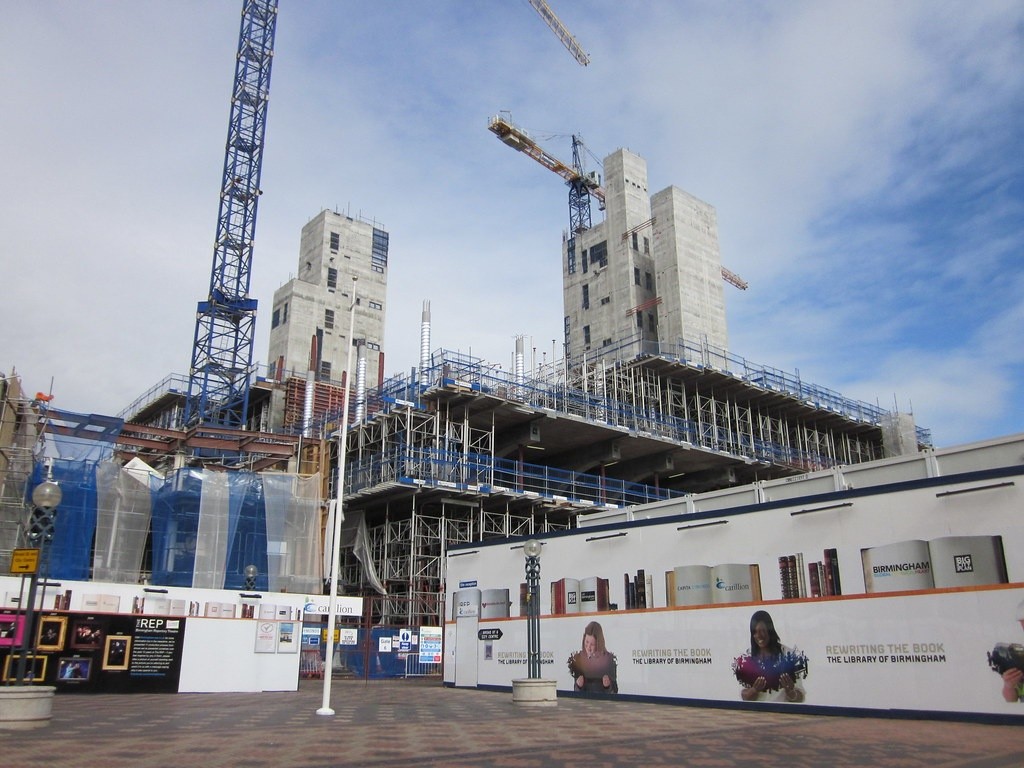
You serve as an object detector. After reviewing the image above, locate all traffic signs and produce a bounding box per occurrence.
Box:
[10,547,39,573]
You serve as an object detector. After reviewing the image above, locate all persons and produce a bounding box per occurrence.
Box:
[567,621,618,694]
[732,610,809,703]
[987,600,1024,703]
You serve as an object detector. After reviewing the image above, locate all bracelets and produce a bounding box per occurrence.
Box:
[786,690,798,701]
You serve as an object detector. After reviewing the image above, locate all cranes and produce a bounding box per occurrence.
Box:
[179,0,593,432]
[485,105,748,291]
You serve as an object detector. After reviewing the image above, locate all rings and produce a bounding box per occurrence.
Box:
[786,684,790,688]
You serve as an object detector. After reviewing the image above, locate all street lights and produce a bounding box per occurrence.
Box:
[525,539,542,680]
[6,457,63,685]
